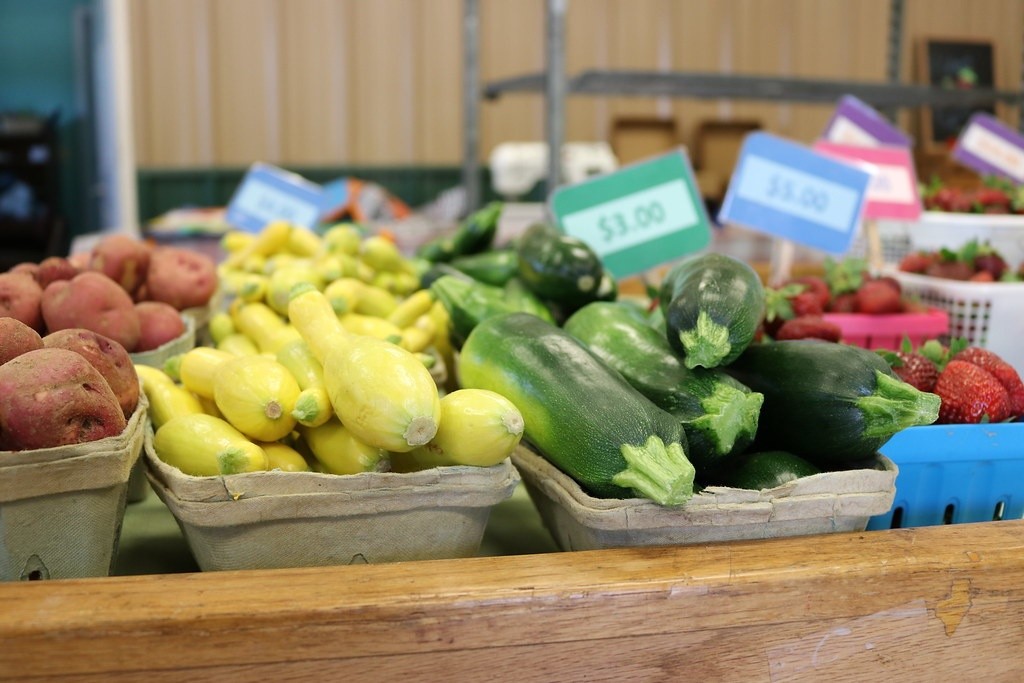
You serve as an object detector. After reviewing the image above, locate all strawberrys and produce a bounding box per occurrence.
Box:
[748,176,1024,424]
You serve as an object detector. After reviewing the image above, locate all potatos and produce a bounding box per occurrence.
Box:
[0,234,217,454]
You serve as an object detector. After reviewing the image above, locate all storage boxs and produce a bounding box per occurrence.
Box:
[0,385,150,581]
[141,416,521,573]
[870,214,1024,384]
[510,443,899,550]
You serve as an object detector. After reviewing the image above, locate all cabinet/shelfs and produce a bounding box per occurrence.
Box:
[461,0,1024,221]
